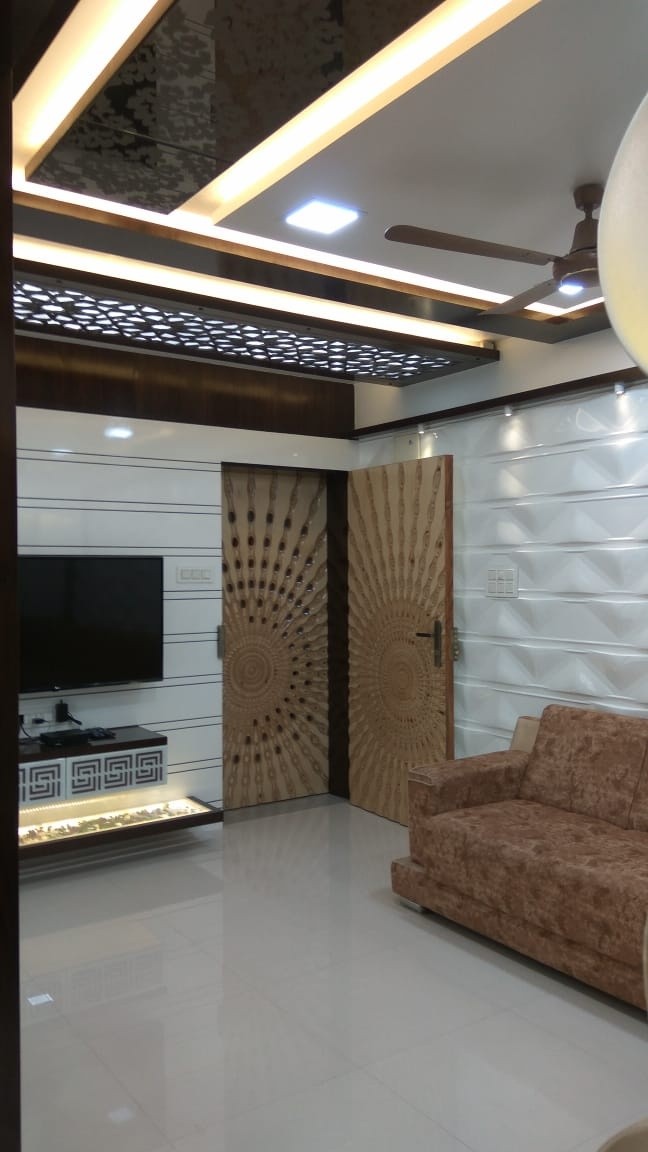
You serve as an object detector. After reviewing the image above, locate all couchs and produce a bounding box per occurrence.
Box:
[390,704,648,1011]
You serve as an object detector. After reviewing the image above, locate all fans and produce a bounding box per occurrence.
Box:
[384,184,600,316]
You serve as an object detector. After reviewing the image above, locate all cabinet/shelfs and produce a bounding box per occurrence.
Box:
[18,726,225,883]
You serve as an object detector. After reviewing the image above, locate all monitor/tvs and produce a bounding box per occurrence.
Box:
[17,554,165,695]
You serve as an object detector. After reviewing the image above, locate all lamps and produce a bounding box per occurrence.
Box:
[614,380,624,395]
[504,404,513,417]
[418,424,424,434]
